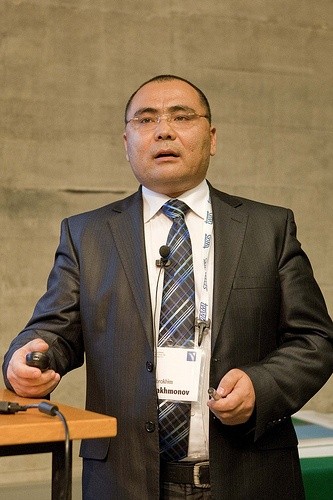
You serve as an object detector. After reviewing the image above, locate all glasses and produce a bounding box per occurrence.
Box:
[125,105,210,132]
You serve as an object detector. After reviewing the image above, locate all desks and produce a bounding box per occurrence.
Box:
[0,387,116,500]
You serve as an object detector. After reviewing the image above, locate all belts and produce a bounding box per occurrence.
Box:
[159,461,211,489]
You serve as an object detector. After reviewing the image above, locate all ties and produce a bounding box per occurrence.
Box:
[157,200,196,465]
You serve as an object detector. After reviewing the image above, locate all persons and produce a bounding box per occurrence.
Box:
[2,74,333,500]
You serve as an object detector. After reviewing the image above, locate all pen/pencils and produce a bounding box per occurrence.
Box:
[208,388,223,401]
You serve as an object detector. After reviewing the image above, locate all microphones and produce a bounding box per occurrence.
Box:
[159,246,170,264]
